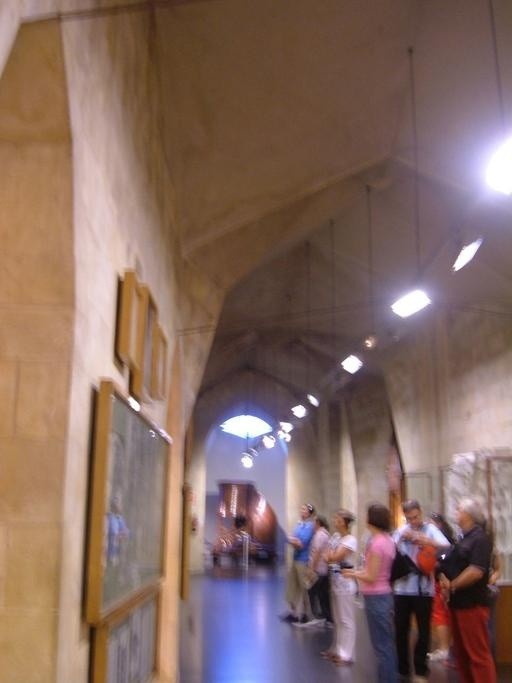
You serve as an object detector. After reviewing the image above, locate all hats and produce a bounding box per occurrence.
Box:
[305,503,315,515]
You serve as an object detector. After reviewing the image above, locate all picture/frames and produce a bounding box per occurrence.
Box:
[89,584,164,683]
[117,272,145,371]
[157,324,168,401]
[131,287,159,404]
[82,379,173,623]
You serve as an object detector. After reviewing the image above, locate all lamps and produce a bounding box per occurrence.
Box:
[290,397,313,420]
[306,370,339,408]
[448,234,485,273]
[339,332,378,376]
[388,260,445,322]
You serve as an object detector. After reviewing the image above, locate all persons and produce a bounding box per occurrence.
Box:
[392,498,452,683]
[339,502,402,683]
[432,497,498,683]
[306,515,334,630]
[320,507,359,669]
[279,503,316,623]
[426,510,462,661]
[428,510,503,669]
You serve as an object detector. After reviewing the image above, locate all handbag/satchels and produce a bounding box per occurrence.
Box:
[392,540,416,582]
